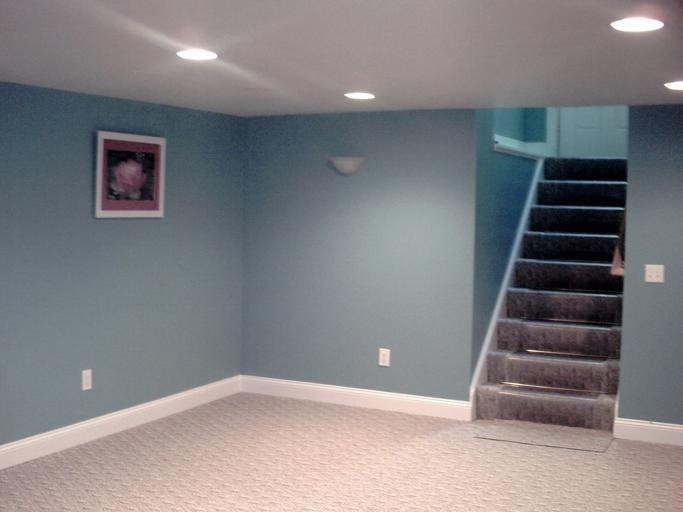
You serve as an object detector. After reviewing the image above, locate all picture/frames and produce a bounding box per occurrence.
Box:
[90,126,166,220]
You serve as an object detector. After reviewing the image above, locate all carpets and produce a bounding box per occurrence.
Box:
[470,418,615,455]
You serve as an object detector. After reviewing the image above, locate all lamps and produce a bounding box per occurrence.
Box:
[326,151,365,176]
